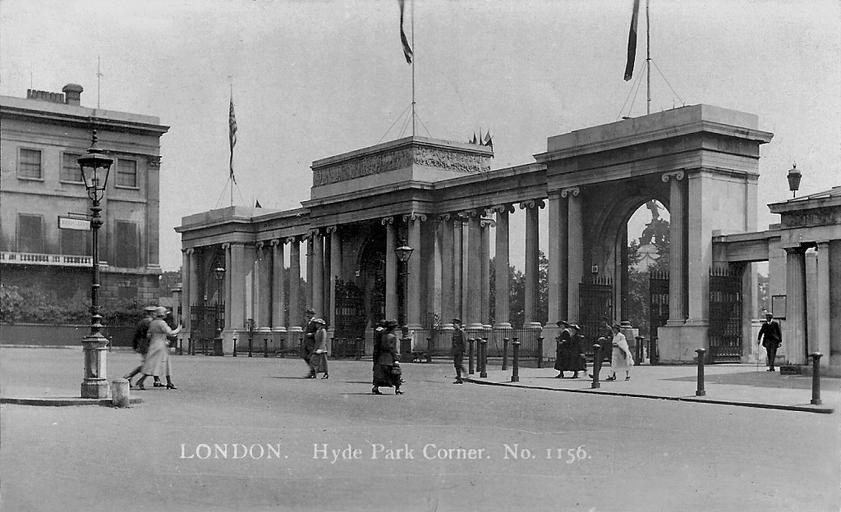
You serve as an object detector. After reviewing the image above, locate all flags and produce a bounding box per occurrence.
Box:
[227,99,237,178]
[468,132,493,149]
[400,1,414,64]
[623,1,643,80]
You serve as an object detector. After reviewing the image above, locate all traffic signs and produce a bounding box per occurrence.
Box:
[56,215,92,232]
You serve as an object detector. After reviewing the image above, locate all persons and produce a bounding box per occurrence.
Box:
[563,324,588,380]
[609,322,636,382]
[589,316,615,381]
[554,320,571,378]
[124,306,167,388]
[757,312,783,373]
[299,308,318,375]
[371,319,405,396]
[134,304,185,392]
[373,320,407,385]
[451,316,468,385]
[303,317,330,380]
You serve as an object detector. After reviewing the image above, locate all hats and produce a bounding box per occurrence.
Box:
[387,320,397,327]
[144,307,156,311]
[155,307,171,316]
[613,325,620,331]
[452,319,461,324]
[557,321,580,331]
[313,318,326,324]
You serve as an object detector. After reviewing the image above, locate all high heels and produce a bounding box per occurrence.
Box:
[372,388,382,394]
[154,382,177,389]
[396,391,404,394]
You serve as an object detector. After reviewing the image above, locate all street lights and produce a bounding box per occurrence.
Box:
[213,266,226,356]
[392,239,414,364]
[75,125,114,400]
[785,159,804,198]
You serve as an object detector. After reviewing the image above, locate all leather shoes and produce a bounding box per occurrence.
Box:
[124,377,145,389]
[454,381,463,384]
[308,374,328,379]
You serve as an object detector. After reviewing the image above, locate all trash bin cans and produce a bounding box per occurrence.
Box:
[214,338,224,356]
